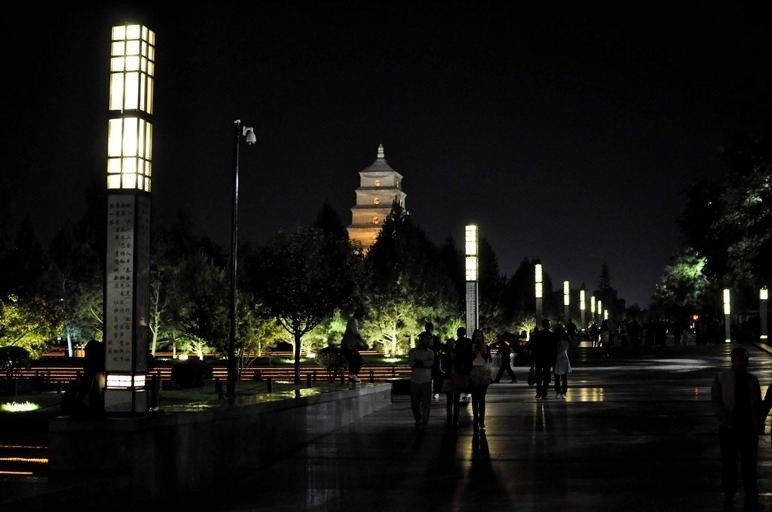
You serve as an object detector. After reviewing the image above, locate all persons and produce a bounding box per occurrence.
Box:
[530,318,576,400]
[83,340,105,376]
[493,336,517,383]
[341,319,367,381]
[408,322,492,429]
[710,348,772,512]
[593,318,673,348]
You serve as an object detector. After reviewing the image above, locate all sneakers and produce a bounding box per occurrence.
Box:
[562,394,566,398]
[348,373,361,381]
[556,393,561,399]
[433,393,440,399]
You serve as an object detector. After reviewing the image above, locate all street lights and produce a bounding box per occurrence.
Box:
[579,289,609,331]
[227,120,257,409]
[102,21,156,420]
[464,222,480,341]
[562,279,572,325]
[723,288,731,343]
[535,262,545,328]
[759,286,769,342]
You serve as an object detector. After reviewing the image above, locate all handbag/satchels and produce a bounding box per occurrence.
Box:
[528,371,536,385]
[471,367,496,385]
[449,373,469,388]
